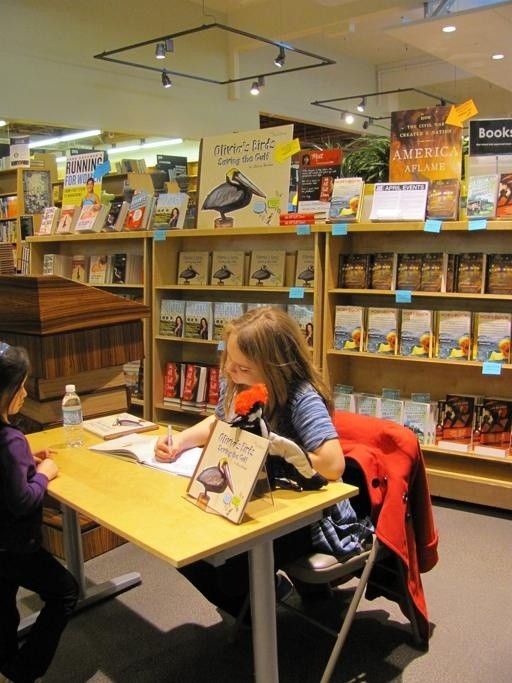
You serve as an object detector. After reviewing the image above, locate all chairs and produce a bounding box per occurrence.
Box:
[227,411,439,683]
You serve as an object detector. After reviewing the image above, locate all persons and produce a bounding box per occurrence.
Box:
[0,341,78,683]
[153,304,376,623]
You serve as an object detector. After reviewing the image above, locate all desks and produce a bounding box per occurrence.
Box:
[24,411,362,683]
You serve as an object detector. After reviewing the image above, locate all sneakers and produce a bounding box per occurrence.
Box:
[276,574,293,607]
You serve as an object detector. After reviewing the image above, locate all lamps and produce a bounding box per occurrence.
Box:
[310,87,459,129]
[27,129,184,162]
[92,22,337,94]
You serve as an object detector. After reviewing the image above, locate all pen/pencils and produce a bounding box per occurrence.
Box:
[168,425,176,463]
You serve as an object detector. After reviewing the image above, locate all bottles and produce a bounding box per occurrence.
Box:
[60,384,85,447]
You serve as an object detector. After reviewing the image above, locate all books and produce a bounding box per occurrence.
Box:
[2,242,144,304]
[186,420,272,525]
[2,104,511,243]
[86,432,206,478]
[332,252,512,461]
[159,249,314,412]
